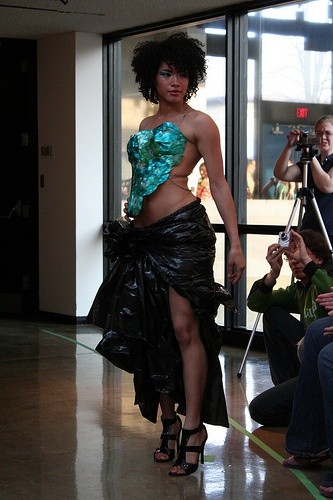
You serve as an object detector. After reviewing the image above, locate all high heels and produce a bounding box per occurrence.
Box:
[154,413,182,462]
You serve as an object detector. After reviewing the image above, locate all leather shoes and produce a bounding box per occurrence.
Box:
[168,424,207,477]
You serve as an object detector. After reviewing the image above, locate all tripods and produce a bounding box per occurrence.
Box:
[236,144,333,378]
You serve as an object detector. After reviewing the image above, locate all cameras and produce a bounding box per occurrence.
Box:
[278,232,291,249]
[300,131,321,146]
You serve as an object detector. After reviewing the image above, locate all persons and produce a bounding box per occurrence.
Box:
[261,159,302,201]
[195,163,211,198]
[242,159,256,198]
[246,229,333,425]
[273,115,333,250]
[283,287,333,495]
[123,33,246,477]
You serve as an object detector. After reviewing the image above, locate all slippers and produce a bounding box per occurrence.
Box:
[282,450,329,468]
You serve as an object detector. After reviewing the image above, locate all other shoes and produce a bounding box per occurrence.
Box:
[320,470,333,494]
